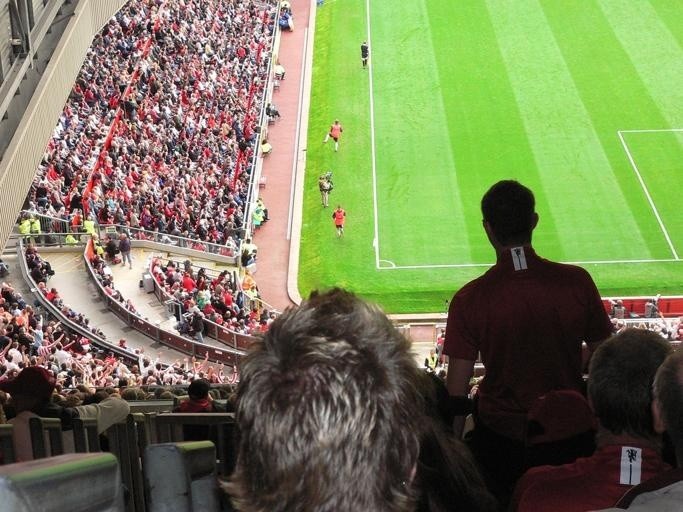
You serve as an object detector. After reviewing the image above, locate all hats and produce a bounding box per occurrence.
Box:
[523,388,600,443]
[0,367,56,400]
[188,381,209,399]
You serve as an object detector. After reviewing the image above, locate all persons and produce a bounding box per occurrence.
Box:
[615,349,682,511]
[15,1,295,273]
[361,40,368,69]
[510,328,674,512]
[219,291,422,512]
[1,173,682,510]
[442,180,616,511]
[323,119,343,152]
